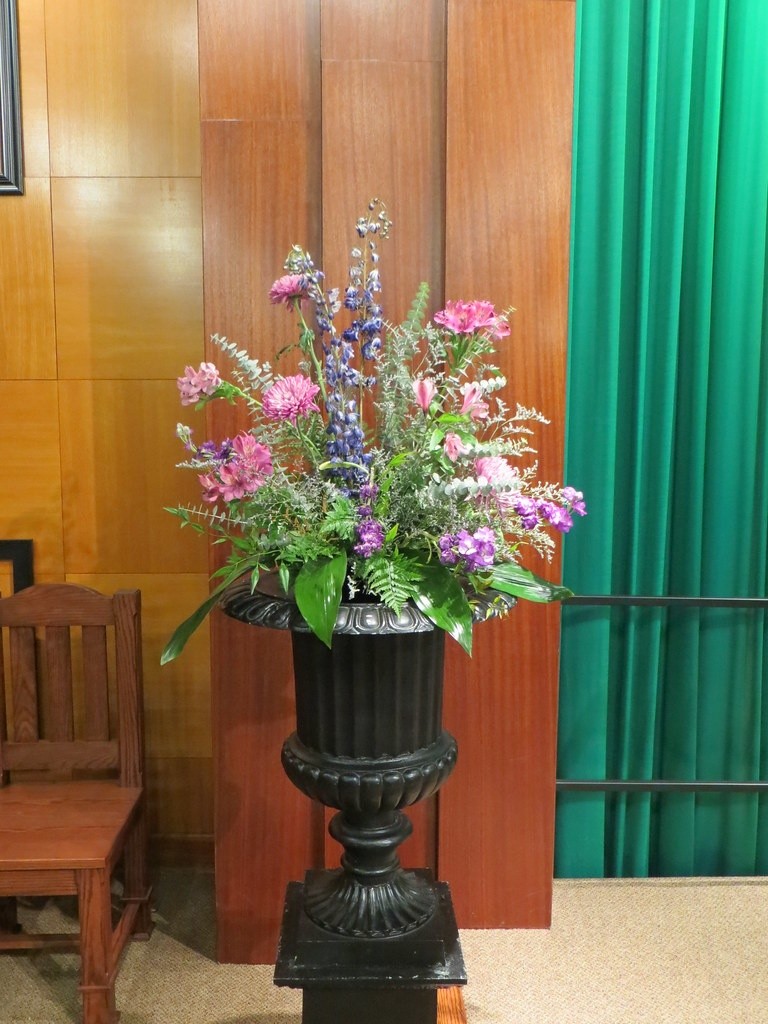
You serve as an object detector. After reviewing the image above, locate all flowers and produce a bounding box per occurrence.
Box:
[145,195,589,672]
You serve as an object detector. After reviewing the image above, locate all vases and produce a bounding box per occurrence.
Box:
[220,567,491,935]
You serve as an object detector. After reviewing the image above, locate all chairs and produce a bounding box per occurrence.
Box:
[0,585,153,1022]
